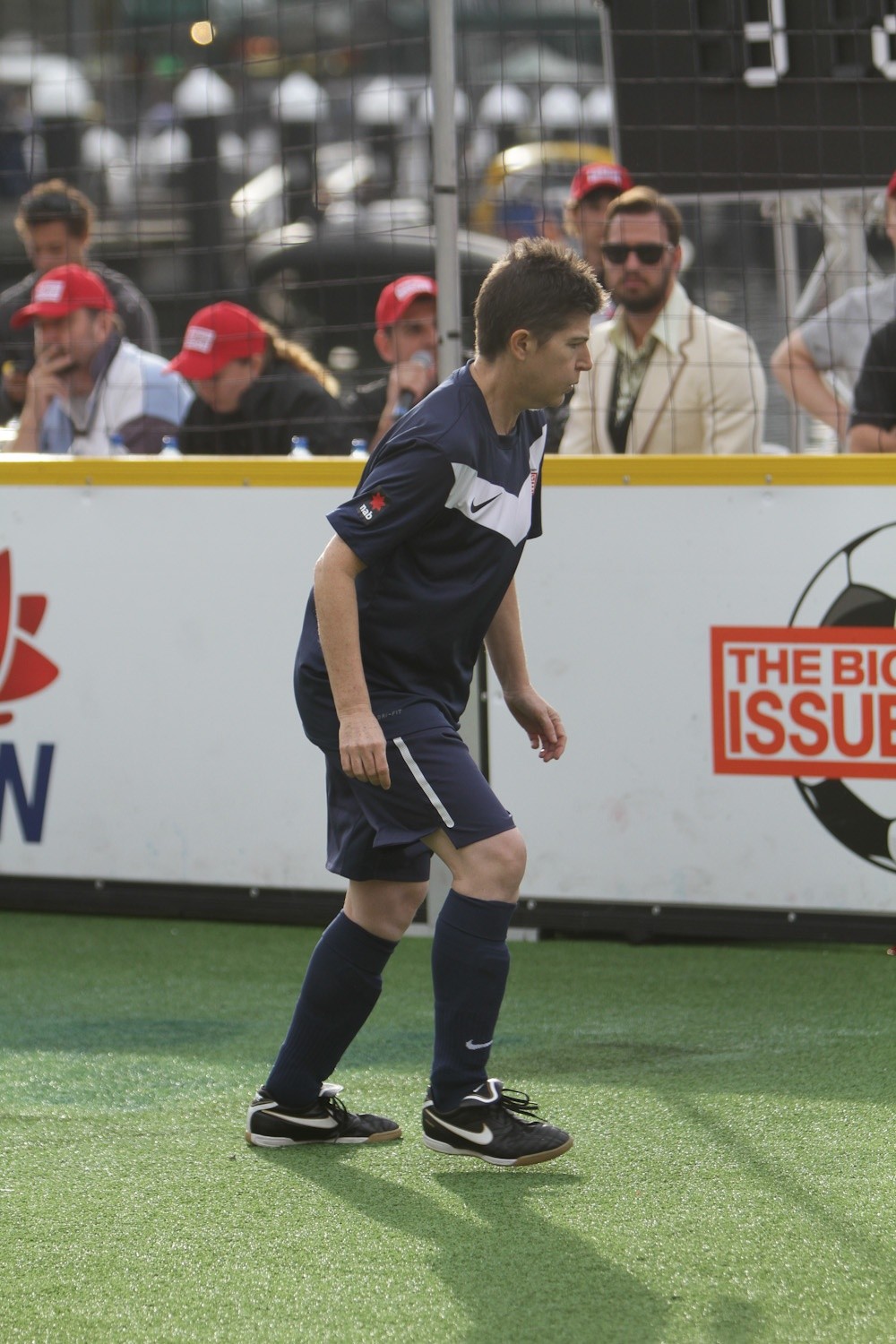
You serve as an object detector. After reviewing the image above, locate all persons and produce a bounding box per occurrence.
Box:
[845,314,895,454]
[546,164,634,453]
[244,231,605,1170]
[159,304,353,460]
[0,174,157,423]
[325,272,480,461]
[550,187,767,457]
[773,177,896,453]
[2,265,195,457]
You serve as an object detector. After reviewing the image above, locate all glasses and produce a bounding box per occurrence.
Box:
[600,242,675,267]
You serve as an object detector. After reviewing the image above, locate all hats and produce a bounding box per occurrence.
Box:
[374,274,437,328]
[9,264,112,330]
[160,302,266,380]
[570,163,631,199]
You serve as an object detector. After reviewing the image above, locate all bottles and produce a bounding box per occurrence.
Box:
[106,434,130,454]
[349,438,369,456]
[157,435,181,454]
[288,435,312,454]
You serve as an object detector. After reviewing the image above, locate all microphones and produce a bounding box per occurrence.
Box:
[391,349,432,420]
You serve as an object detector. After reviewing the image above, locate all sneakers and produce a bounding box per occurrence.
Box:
[422,1079,572,1168]
[244,1085,404,1149]
